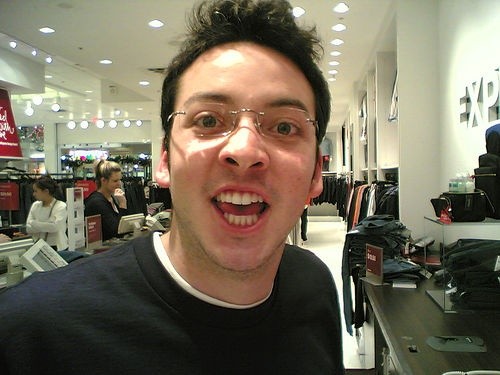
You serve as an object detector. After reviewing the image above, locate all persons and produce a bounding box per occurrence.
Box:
[82,158,129,243]
[25,174,70,253]
[0,0,347,375]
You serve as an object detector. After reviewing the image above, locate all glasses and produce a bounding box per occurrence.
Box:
[163,103,322,140]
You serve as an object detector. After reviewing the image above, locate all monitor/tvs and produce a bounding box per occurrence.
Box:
[20,239,70,274]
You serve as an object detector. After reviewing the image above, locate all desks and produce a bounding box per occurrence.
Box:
[362,272,500,375]
